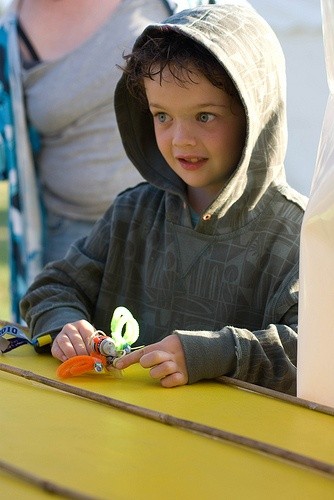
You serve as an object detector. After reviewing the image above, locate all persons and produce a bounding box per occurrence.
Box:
[0,0,251,347]
[20,3,309,388]
